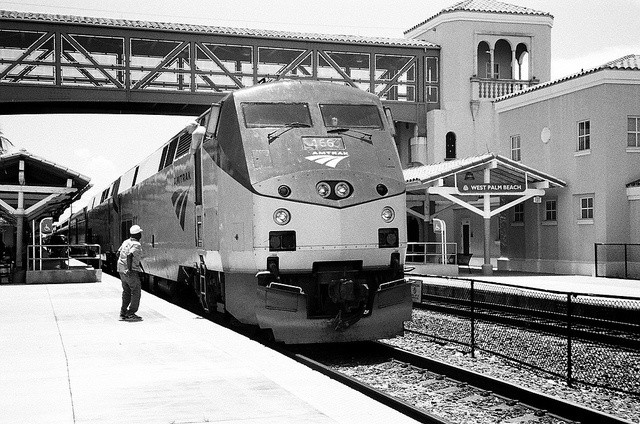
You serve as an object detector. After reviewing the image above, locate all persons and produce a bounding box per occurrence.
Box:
[115,224,143,321]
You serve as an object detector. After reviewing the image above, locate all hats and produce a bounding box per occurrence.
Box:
[130,224,143,235]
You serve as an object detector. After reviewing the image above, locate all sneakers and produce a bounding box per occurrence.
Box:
[119,313,126,321]
[125,313,142,321]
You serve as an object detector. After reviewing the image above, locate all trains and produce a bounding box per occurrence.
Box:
[55,80,411,344]
